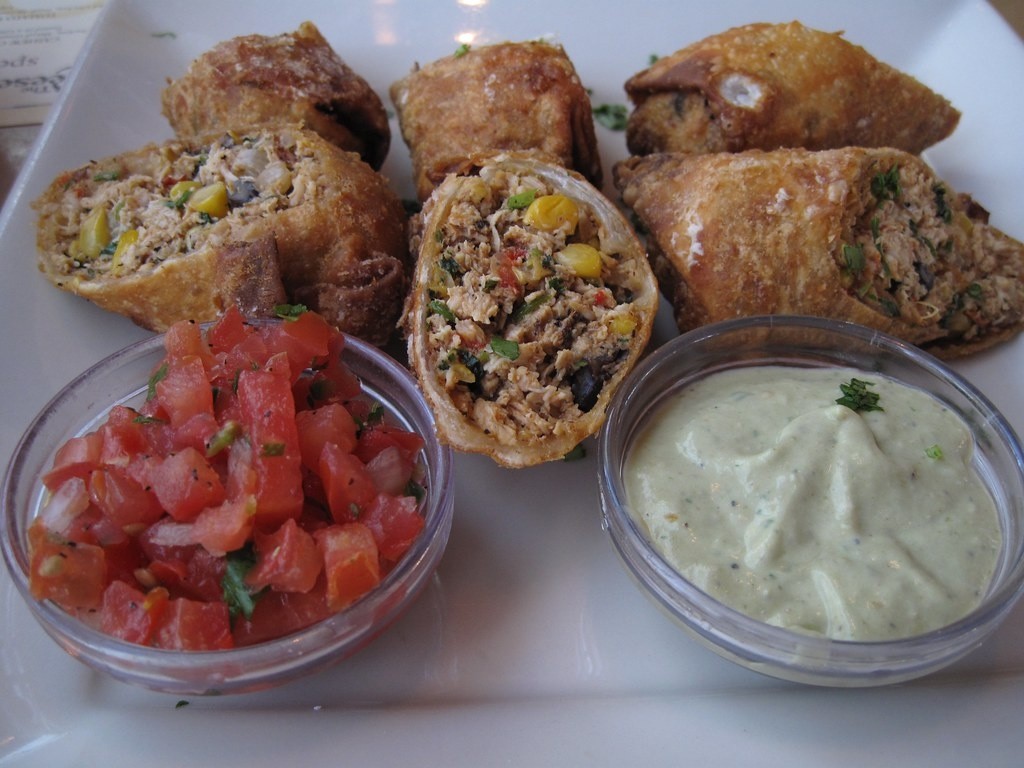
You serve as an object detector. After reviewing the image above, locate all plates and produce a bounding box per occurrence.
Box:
[0,0,1021,767]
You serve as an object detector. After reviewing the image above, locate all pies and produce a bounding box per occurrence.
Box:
[29,22,1023,466]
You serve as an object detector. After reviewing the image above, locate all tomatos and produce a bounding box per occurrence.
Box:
[21,307,431,697]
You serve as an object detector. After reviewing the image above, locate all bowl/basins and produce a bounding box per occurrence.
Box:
[1,317,455,693]
[589,313,1024,691]
